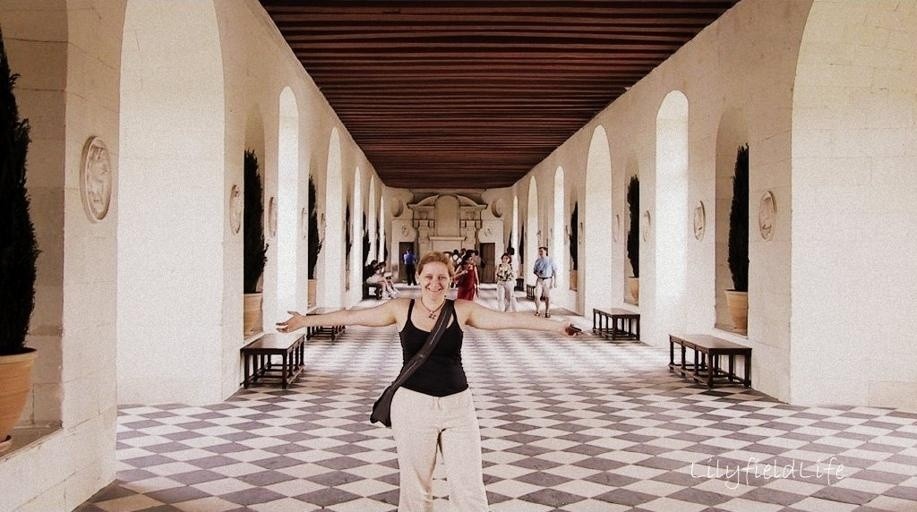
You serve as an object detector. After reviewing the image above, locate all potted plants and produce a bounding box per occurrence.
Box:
[626,174,639,304]
[568,201,577,291]
[346,204,352,290]
[724,141,748,336]
[244,147,268,342]
[0,24,43,456]
[519,225,524,276]
[309,175,322,308]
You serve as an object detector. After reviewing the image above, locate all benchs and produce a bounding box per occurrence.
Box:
[527,284,543,300]
[240,333,306,389]
[306,307,346,341]
[667,333,752,392]
[593,308,640,341]
[363,283,382,300]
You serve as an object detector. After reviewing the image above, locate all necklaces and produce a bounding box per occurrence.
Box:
[420,296,446,321]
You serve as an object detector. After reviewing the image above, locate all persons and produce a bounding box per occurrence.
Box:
[533,246,557,318]
[276,250,582,512]
[495,253,518,313]
[403,247,421,289]
[442,248,482,301]
[504,246,519,312]
[364,260,400,300]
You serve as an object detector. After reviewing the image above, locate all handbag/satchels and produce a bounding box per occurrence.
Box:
[368,384,397,431]
